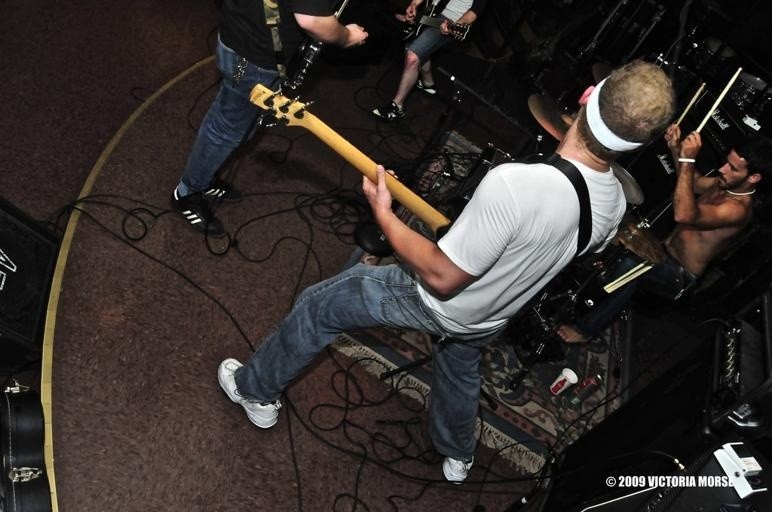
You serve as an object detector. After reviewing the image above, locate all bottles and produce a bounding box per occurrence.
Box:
[552,378,567,394]
[568,373,602,404]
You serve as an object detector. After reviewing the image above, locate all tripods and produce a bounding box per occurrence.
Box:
[506,274,596,392]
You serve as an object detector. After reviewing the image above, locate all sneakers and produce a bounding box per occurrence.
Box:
[371,99,409,121]
[202,178,243,203]
[171,187,226,238]
[415,77,438,95]
[217,357,282,430]
[442,452,476,487]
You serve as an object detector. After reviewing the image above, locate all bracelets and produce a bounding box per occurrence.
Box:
[678,156,696,164]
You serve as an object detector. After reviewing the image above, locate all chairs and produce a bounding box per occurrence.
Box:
[636,268,726,318]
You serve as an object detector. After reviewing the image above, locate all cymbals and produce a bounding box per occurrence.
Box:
[617,210,666,264]
[528,94,572,142]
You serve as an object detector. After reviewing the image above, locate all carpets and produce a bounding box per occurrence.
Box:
[329,113,635,491]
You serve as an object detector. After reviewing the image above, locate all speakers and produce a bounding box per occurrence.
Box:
[0,193,61,356]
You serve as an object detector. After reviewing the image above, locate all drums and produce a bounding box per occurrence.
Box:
[693,35,735,80]
[729,73,772,119]
[611,163,643,207]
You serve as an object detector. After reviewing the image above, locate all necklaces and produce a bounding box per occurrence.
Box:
[724,188,756,196]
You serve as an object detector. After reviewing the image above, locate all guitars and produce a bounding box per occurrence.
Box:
[248,85,454,241]
[400,0,472,40]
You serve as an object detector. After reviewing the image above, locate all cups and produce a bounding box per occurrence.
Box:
[550,368,579,395]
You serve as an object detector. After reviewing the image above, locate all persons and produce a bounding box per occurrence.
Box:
[216,58,678,486]
[373,0,486,123]
[554,122,770,346]
[169,0,372,239]
